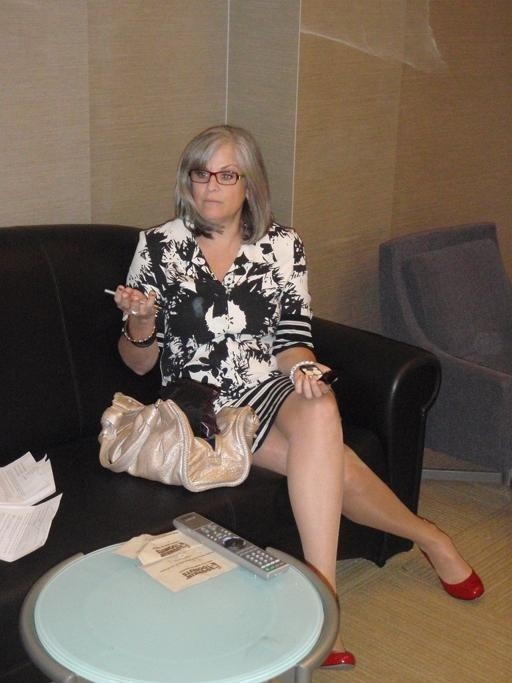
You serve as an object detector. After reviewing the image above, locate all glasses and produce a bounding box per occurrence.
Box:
[189,168,245,184]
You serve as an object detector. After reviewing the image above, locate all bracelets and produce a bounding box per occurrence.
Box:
[289,359,316,386]
[122,320,158,350]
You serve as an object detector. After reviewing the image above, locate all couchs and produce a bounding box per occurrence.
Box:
[1,223,427,672]
[378,217,511,492]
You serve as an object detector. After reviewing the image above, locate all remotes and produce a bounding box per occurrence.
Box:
[172,512,290,580]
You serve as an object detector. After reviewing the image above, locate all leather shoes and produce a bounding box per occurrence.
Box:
[318,649,356,671]
[418,514,486,600]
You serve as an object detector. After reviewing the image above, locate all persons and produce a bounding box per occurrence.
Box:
[114,120,488,674]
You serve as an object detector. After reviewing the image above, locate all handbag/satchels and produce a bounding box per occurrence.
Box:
[97,391,260,493]
[159,379,220,438]
